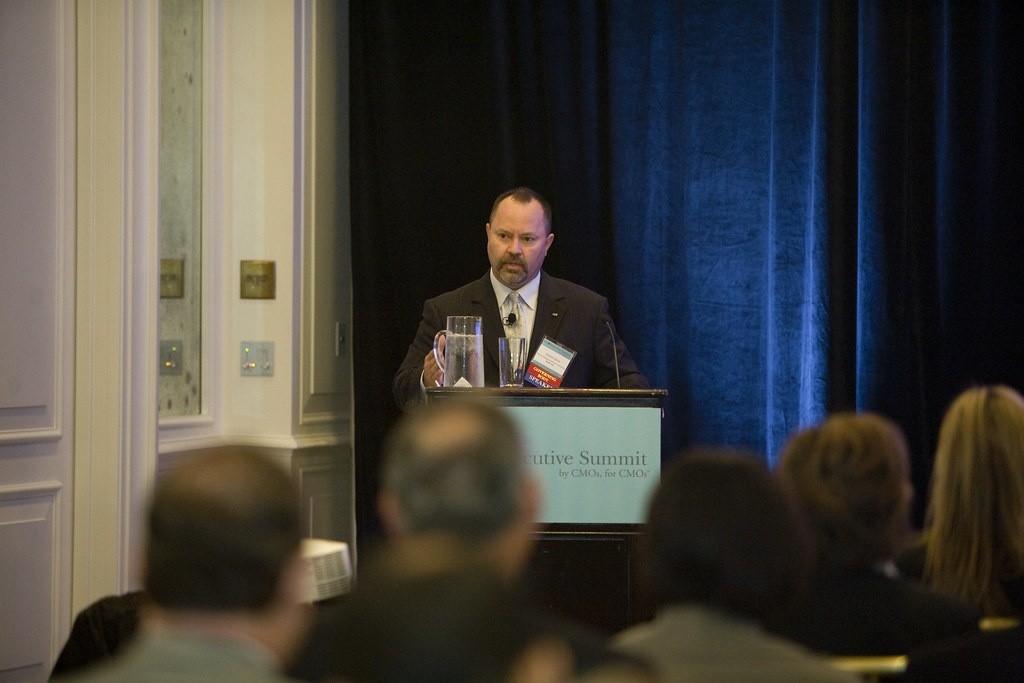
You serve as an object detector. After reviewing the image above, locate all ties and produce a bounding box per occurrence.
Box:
[507,292,528,376]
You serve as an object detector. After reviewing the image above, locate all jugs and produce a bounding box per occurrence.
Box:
[433,315,486,387]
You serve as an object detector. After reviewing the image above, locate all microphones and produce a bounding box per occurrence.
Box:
[600,314,620,389]
[508,312,516,326]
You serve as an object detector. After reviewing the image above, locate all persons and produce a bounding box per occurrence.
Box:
[49,388,1024,682]
[391,189,649,414]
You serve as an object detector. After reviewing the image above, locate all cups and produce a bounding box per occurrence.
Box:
[497,337,527,388]
